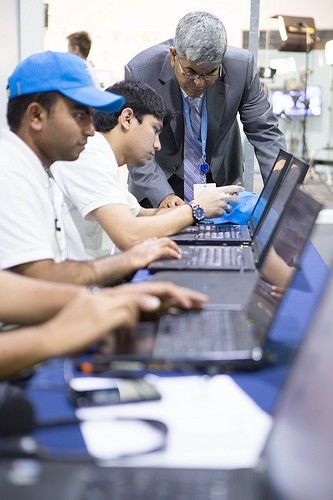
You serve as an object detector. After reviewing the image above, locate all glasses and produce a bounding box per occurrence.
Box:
[176,59,222,80]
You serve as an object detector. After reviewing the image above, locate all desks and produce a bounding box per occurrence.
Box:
[18,192,333,461]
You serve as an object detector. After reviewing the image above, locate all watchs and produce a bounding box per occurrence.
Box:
[189,202,205,226]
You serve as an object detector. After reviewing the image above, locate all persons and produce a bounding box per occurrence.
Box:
[0,51,180,288]
[0,270,208,441]
[124,12,287,208]
[66,32,91,61]
[50,79,245,258]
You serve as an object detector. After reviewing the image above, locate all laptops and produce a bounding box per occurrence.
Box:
[147,156,311,272]
[170,148,292,245]
[75,188,323,375]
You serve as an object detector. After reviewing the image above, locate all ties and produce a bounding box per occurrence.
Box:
[184,97,207,203]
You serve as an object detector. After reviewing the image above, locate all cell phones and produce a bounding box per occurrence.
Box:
[72,380,163,408]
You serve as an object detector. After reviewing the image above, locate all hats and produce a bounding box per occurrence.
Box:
[7,51,124,114]
